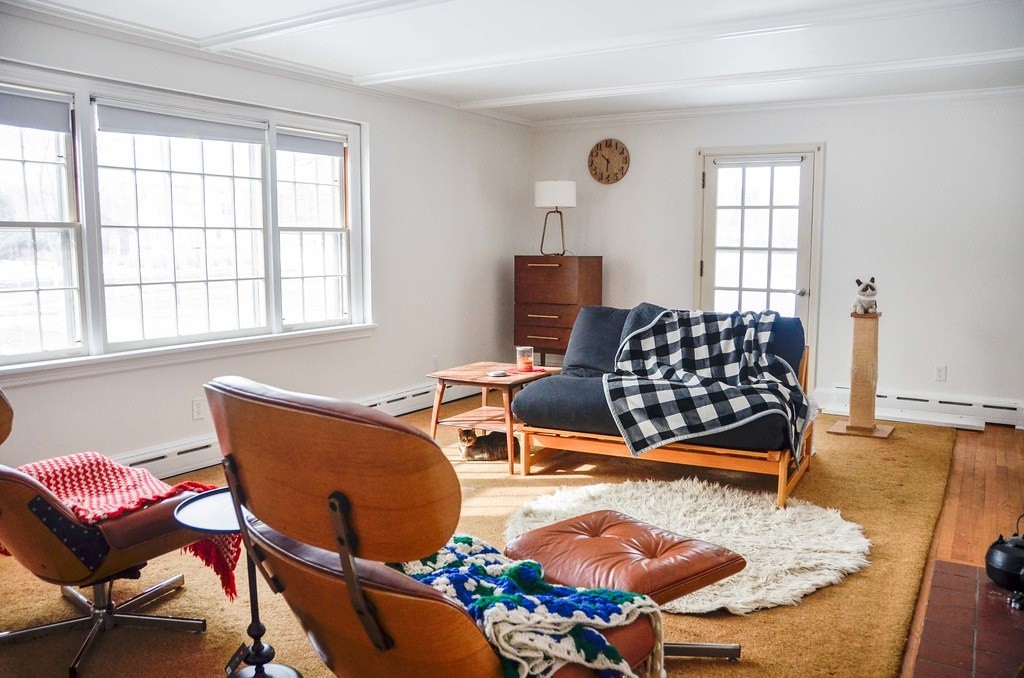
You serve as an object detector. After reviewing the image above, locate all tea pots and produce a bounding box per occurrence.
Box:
[985,514,1024,591]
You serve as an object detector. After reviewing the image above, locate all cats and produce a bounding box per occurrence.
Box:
[457,428,520,461]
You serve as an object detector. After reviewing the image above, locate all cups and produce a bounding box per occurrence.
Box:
[516,346,533,372]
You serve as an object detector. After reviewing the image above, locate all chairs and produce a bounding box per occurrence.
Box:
[202,378,748,677]
[0,390,221,674]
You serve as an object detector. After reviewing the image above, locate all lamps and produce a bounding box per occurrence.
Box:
[533,179,578,256]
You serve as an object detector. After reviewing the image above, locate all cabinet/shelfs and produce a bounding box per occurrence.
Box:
[512,253,603,366]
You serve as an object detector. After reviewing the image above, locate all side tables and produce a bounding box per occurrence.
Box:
[426,360,563,476]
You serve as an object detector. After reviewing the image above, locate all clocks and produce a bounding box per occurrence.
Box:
[588,138,631,186]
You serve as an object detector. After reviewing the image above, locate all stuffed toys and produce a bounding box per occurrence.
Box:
[853,276,879,315]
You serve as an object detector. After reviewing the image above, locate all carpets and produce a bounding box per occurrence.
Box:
[0,371,960,678]
[503,477,874,613]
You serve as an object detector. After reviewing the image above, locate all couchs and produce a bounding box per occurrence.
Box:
[514,302,816,507]
